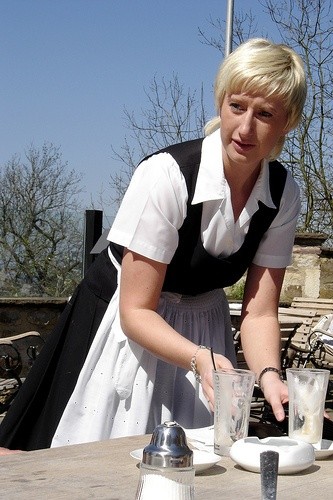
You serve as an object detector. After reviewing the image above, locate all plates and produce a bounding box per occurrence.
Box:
[128,447,222,473]
[282,435,333,460]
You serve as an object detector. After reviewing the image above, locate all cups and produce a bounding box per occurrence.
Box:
[212,368,256,457]
[285,367,330,450]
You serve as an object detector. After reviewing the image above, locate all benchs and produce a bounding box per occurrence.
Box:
[221,296,333,425]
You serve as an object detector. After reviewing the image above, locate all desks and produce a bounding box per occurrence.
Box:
[0,426,333,500]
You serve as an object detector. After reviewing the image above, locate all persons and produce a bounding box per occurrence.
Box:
[0,38,308,452]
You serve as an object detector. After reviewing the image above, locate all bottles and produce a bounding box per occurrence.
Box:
[134,420,194,500]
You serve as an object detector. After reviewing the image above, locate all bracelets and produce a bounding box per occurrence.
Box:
[257,367,284,389]
[190,343,213,382]
